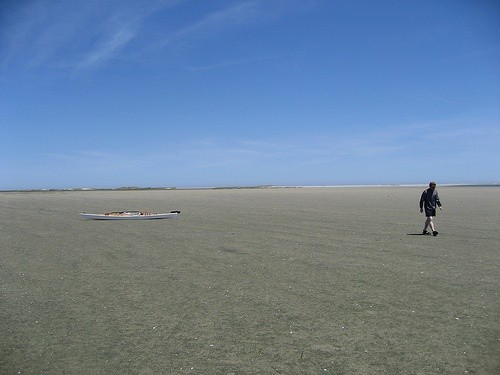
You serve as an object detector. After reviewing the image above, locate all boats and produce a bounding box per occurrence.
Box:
[79,210,180,219]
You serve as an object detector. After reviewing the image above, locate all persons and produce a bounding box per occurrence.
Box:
[420,182,442,236]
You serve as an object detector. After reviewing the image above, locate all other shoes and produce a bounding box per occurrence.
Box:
[422,230,431,235]
[433,230,438,236]
